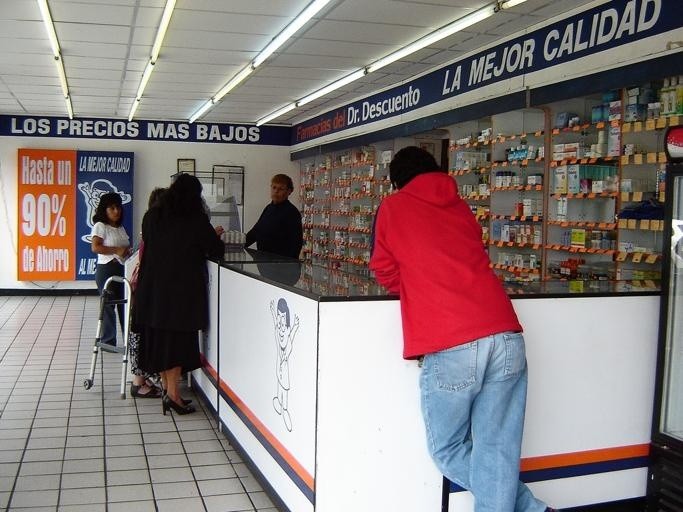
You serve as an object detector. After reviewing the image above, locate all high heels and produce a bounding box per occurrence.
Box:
[162,389,196,415]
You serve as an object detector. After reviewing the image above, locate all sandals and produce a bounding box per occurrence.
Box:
[131,382,162,398]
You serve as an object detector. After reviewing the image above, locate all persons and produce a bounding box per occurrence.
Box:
[244,174,303,259]
[368,146,559,512]
[131,174,225,415]
[90,191,134,352]
[127,187,223,400]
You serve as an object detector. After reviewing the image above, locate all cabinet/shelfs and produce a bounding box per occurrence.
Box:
[297,77,683,281]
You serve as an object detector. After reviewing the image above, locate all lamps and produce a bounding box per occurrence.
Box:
[34,0,529,127]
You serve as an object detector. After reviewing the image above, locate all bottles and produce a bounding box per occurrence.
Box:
[513,202,522,216]
[222,230,245,243]
[646,76,683,119]
[655,163,665,203]
[493,144,537,188]
[576,261,615,281]
[590,230,604,250]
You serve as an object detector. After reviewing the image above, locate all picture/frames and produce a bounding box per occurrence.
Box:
[176,158,195,176]
[211,164,245,206]
